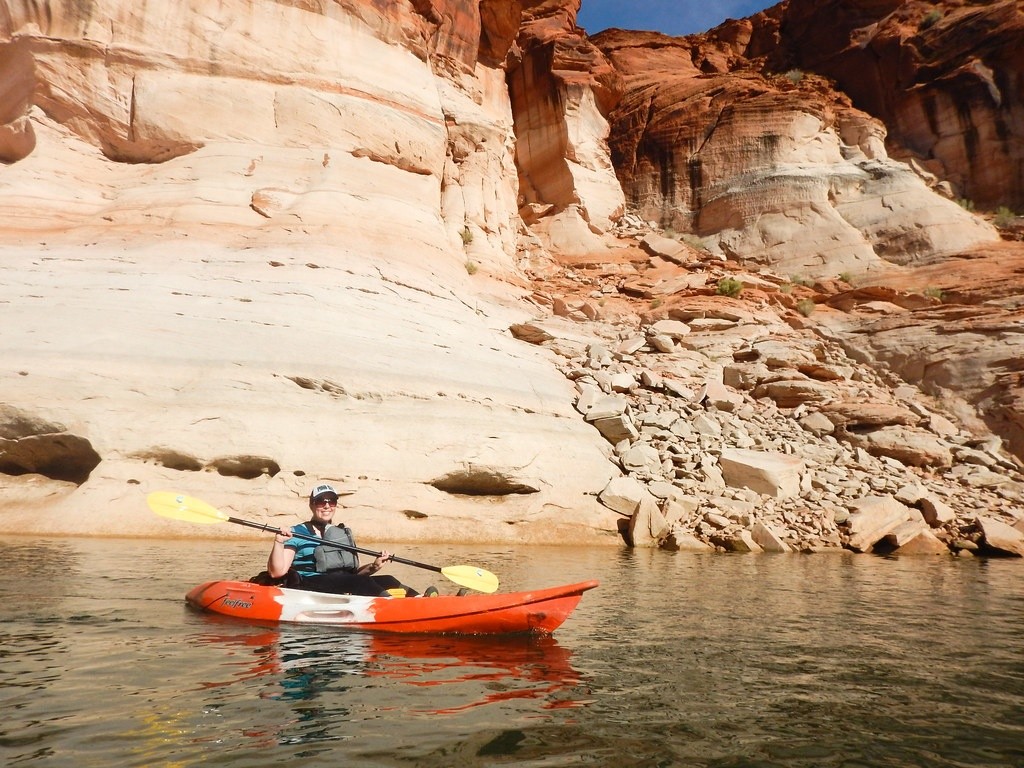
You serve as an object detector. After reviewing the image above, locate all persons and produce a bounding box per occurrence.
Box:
[267,485,467,597]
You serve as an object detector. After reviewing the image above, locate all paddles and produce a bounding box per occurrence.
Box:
[145,488,501,595]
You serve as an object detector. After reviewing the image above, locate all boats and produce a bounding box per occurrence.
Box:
[179,578,600,636]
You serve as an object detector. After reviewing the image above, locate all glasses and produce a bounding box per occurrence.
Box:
[315,497,337,508]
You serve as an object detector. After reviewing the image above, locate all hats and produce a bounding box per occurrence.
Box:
[310,484,339,499]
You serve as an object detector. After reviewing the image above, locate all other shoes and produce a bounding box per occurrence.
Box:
[424,586,439,597]
[456,588,468,596]
